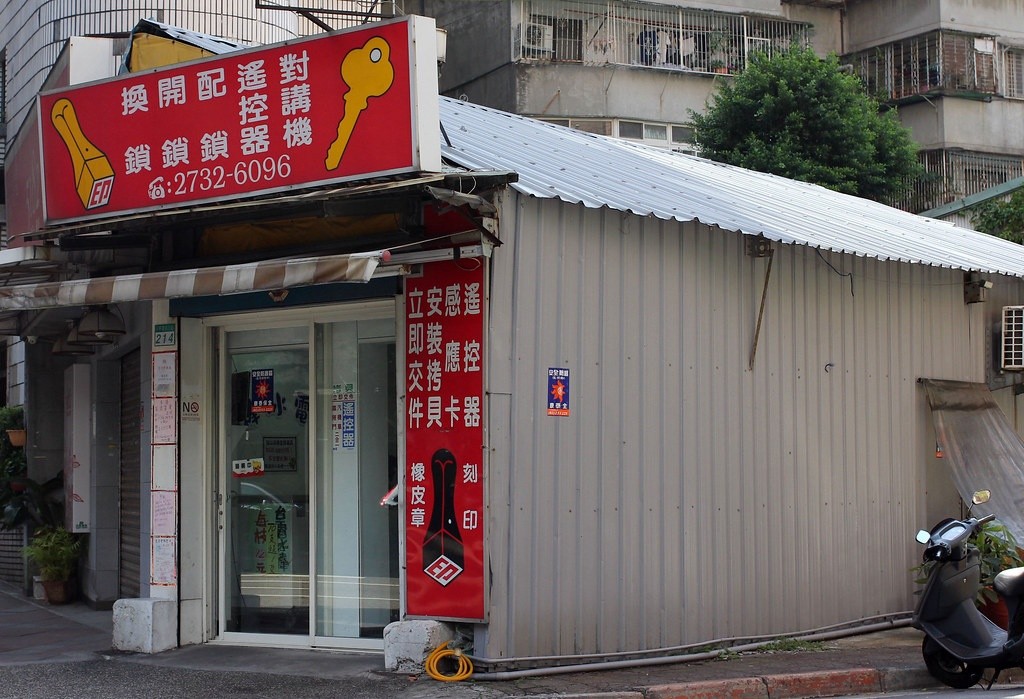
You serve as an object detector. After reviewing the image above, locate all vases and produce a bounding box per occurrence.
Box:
[729,69,735,75]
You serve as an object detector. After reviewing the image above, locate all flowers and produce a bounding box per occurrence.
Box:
[726,64,736,70]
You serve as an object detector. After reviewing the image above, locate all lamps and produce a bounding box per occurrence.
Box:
[76,302,127,337]
[52,334,96,356]
[66,325,114,348]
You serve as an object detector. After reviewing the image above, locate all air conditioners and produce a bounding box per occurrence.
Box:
[518,21,554,52]
[1001,305,1024,372]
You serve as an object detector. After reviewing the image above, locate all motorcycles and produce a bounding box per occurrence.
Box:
[912,489,1024,689]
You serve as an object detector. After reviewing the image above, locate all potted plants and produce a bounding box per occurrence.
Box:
[18,521,87,605]
[1,470,66,601]
[692,63,707,72]
[908,518,1024,633]
[2,406,26,447]
[4,450,27,491]
[712,60,728,74]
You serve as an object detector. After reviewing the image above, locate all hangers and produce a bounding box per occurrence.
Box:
[594,21,614,46]
[644,26,695,39]
